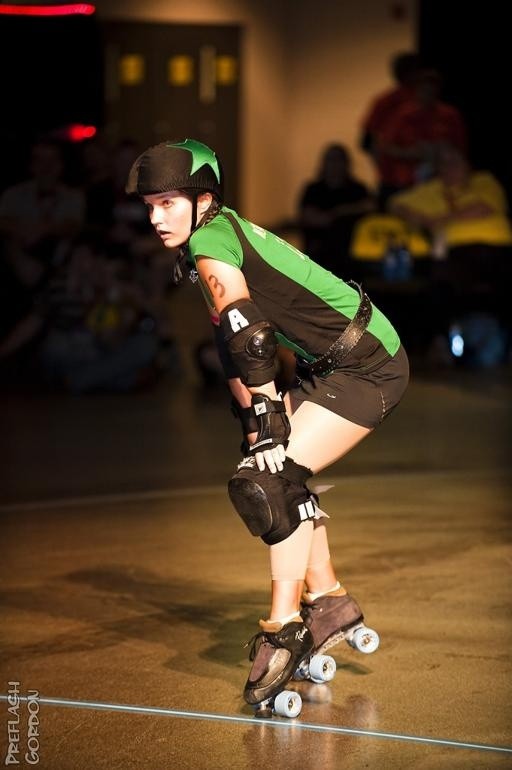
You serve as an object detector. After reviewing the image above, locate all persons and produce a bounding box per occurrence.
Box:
[121,138,413,706]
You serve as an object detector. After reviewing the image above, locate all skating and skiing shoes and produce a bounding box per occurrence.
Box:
[293,578,380,681]
[242,615,337,719]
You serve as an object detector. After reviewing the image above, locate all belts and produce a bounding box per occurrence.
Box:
[292,280,372,378]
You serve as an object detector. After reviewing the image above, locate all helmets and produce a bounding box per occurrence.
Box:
[125,136,224,196]
